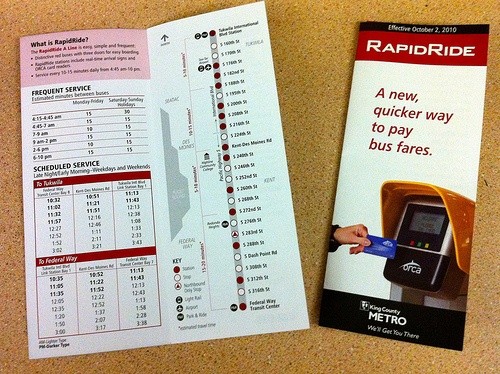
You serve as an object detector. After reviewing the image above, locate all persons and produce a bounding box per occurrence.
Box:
[327,221,373,255]
